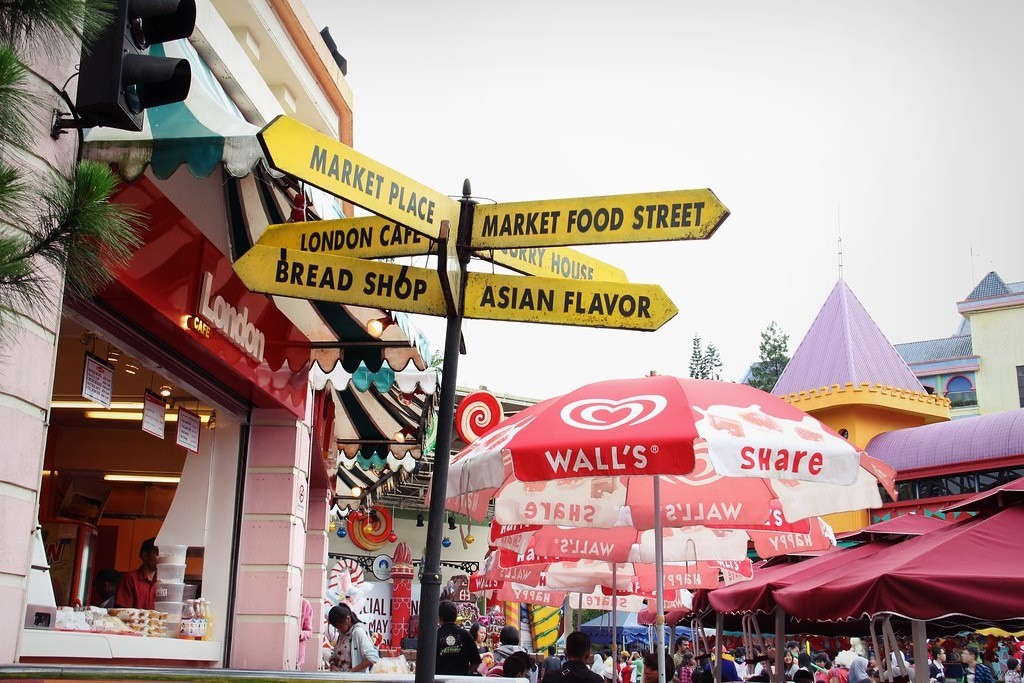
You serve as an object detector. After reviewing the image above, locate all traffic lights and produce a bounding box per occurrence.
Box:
[74,0,199,135]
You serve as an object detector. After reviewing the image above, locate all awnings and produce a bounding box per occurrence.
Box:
[330,463,414,512]
[308,353,437,472]
[82,24,444,375]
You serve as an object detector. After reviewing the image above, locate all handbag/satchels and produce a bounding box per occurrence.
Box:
[830,668,839,683]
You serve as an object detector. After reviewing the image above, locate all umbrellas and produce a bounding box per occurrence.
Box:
[425,369,896,683]
[637,474,1024,683]
[486,514,837,683]
[468,549,753,633]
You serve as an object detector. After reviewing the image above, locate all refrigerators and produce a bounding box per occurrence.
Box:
[41,516,98,608]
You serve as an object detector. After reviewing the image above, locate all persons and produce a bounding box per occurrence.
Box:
[436,601,482,675]
[114,537,159,609]
[327,604,380,673]
[298,599,313,665]
[93,566,119,608]
[470,616,1024,683]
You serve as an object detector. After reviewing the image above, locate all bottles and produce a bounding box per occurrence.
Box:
[179,598,212,640]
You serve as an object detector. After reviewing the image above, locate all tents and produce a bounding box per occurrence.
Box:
[581,609,698,653]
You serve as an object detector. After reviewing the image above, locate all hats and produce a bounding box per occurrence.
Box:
[712,644,729,655]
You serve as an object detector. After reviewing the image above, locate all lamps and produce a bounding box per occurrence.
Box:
[78,330,96,346]
[160,379,171,396]
[124,358,143,375]
[368,509,381,524]
[108,347,120,361]
[415,514,424,527]
[448,517,457,530]
[86,411,212,422]
[367,316,398,339]
[397,388,425,406]
[100,472,181,483]
[51,401,175,410]
[395,428,408,443]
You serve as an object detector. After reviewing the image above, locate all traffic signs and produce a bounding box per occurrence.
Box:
[438,219,462,317]
[469,237,631,291]
[254,114,460,242]
[230,244,452,319]
[468,187,733,250]
[461,272,682,335]
[248,215,438,261]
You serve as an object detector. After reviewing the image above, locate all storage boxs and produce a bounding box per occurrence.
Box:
[379,636,418,661]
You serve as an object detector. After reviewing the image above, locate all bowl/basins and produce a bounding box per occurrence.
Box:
[157,564,186,582]
[158,544,188,565]
[154,583,197,638]
